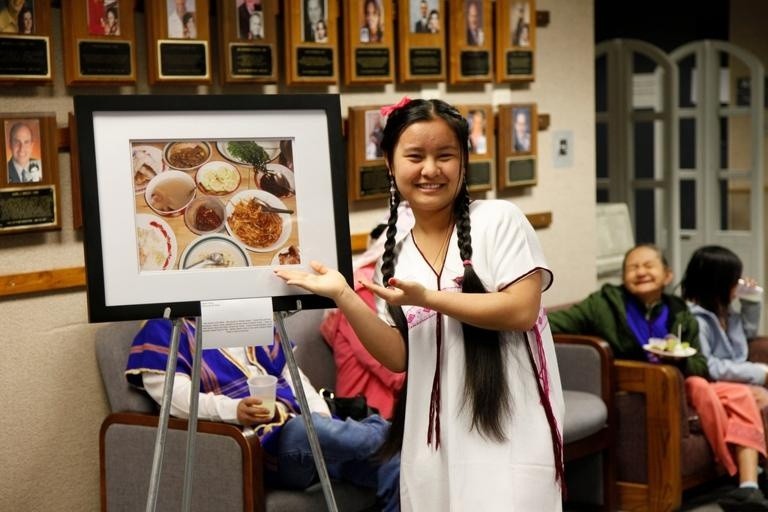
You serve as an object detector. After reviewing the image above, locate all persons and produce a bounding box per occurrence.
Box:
[321,222,421,426]
[0,2,552,240]
[123,309,401,512]
[545,246,767,512]
[673,245,767,434]
[271,98,566,512]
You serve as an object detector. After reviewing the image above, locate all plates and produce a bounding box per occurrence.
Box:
[132,146,169,197]
[223,189,293,253]
[642,340,699,359]
[270,246,301,266]
[136,214,176,271]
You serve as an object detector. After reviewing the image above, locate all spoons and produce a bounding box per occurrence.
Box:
[253,197,294,215]
[181,252,223,270]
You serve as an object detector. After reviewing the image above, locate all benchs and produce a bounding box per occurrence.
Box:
[613,332,767,510]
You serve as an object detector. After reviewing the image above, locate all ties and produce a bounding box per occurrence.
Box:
[22,169,28,182]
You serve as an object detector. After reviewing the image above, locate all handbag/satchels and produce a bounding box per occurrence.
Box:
[325,396,367,420]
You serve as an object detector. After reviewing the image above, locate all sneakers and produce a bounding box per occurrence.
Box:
[713,466,768,512]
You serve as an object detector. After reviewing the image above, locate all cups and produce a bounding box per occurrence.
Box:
[734,278,763,302]
[247,374,279,422]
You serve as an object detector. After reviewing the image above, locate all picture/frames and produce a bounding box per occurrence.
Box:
[0,112,61,237]
[0,0,54,84]
[73,92,352,323]
[61,1,138,88]
[348,100,540,202]
[146,1,212,86]
[67,113,83,228]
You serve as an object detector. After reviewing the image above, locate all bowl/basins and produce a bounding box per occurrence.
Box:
[177,232,253,272]
[194,161,242,198]
[254,163,296,201]
[144,171,198,218]
[161,141,213,171]
[215,140,282,167]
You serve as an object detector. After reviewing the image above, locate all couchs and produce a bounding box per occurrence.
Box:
[93,308,620,512]
